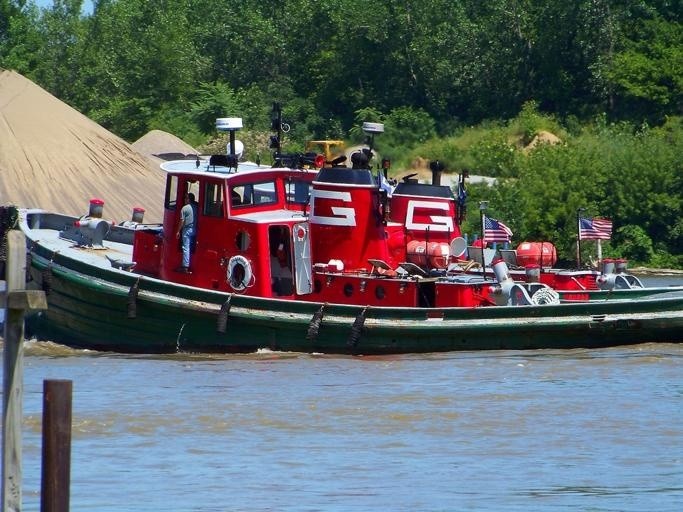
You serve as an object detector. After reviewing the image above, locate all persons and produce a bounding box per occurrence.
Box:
[175,193,199,273]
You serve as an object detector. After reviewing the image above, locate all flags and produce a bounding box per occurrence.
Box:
[579,217,613,240]
[482,214,514,244]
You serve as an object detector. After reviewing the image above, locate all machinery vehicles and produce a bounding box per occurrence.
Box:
[298,139,347,170]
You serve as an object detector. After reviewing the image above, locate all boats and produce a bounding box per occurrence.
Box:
[13,101,682,356]
